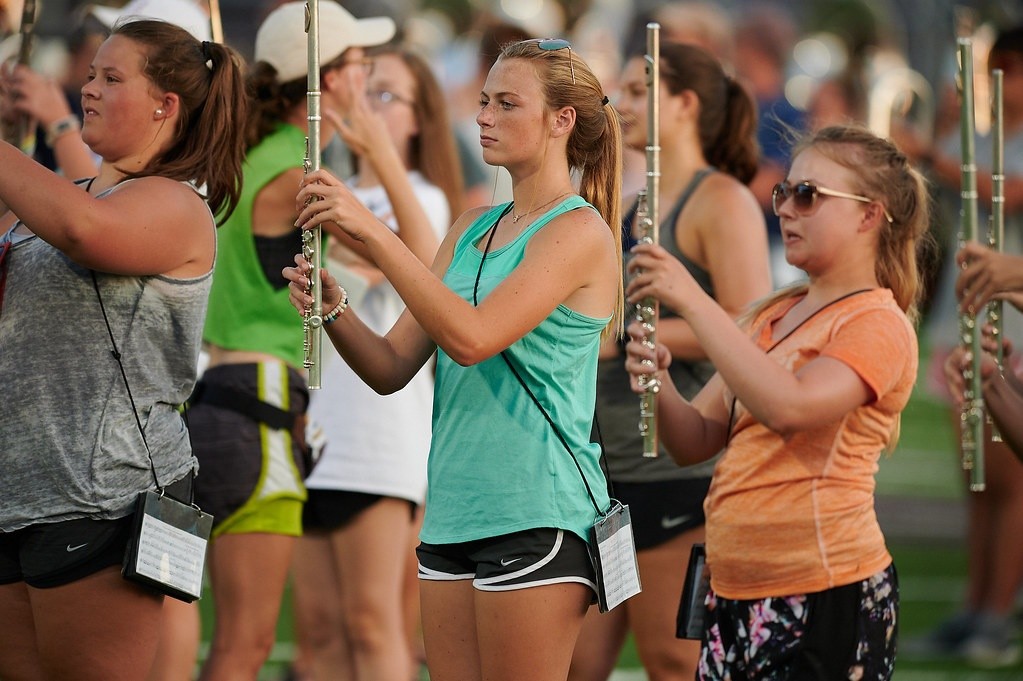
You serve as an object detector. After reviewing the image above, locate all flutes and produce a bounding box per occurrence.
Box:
[1,1,35,147]
[957,38,1016,494]
[300,1,325,389]
[635,24,662,457]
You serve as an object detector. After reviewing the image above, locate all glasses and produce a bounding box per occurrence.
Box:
[333,57,375,78]
[772,180,894,223]
[370,90,415,110]
[538,36,574,86]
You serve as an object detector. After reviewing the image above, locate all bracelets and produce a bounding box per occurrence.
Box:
[321,285,348,323]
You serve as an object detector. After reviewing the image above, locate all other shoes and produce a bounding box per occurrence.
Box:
[898,610,1023,667]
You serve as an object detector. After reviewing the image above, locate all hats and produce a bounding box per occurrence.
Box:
[255,2,395,85]
[90,0,213,69]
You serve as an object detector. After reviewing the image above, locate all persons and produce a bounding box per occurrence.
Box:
[202,0,1023,681]
[0,20,249,681]
[0,0,224,181]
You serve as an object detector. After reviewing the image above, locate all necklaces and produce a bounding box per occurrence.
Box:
[512,191,577,223]
